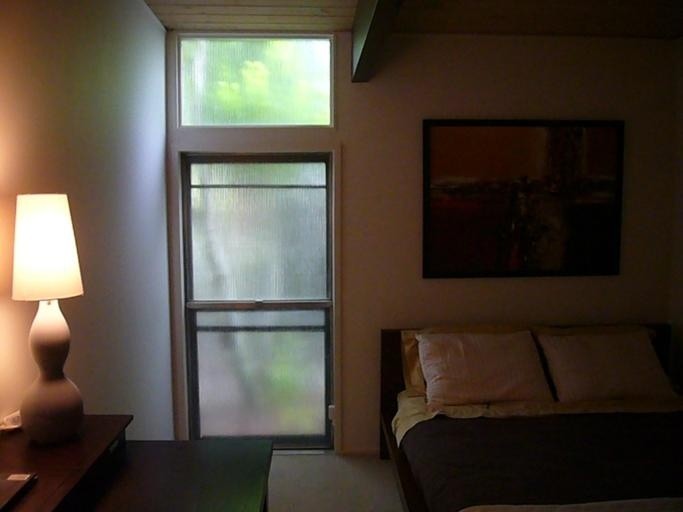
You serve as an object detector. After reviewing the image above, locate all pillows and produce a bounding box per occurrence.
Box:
[401,325,678,413]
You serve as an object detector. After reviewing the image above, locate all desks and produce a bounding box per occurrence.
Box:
[0,414,134,512]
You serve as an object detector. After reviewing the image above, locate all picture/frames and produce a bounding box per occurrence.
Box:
[423,119,625,276]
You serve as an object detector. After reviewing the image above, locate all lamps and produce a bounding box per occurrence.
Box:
[11,194,84,446]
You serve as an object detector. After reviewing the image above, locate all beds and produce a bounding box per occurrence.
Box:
[380,329,683,512]
